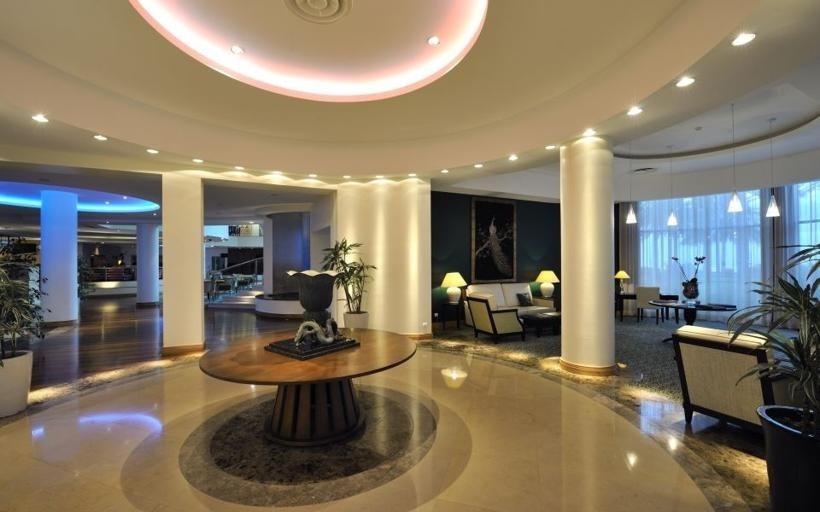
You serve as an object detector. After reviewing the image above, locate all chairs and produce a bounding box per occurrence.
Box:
[467,297,525,344]
[637,287,660,324]
[671,324,798,432]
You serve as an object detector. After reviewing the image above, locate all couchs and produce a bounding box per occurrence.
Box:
[464,283,556,329]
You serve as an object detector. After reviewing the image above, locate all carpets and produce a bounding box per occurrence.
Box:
[415,317,799,403]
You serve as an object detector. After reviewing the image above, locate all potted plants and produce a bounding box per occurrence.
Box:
[319,238,376,328]
[726,244,819,512]
[0,232,53,419]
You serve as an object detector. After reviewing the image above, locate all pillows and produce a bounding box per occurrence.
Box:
[516,292,533,307]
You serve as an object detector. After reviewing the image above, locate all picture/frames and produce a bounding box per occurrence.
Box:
[470,197,518,283]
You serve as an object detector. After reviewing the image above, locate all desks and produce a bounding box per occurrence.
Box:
[616,294,680,324]
[199,329,417,447]
[435,304,465,329]
[650,300,737,342]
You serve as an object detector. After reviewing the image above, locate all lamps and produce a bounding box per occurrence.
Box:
[765,122,781,218]
[626,138,636,224]
[615,271,631,294]
[666,148,678,226]
[535,270,560,297]
[441,272,467,305]
[728,103,743,213]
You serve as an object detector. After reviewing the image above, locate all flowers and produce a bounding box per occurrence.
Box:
[671,254,706,282]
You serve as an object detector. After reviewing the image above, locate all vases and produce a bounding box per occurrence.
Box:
[683,282,698,299]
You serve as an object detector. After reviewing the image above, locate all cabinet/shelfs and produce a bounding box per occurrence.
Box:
[85,266,125,281]
